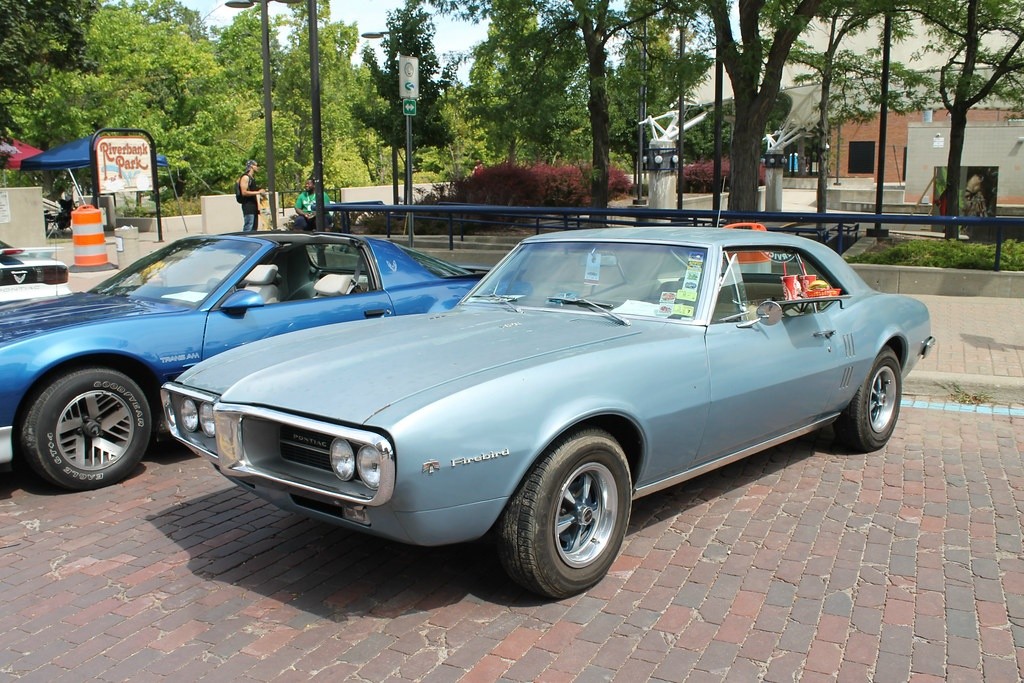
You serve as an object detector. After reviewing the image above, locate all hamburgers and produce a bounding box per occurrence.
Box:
[807,280,829,291]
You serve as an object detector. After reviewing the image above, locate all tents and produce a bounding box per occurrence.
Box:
[18,133,189,233]
[0,135,86,205]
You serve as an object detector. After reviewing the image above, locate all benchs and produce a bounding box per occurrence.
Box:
[656,281,785,323]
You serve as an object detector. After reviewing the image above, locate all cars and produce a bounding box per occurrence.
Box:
[0,230,532,493]
[163,227,936,604]
[0,238,70,303]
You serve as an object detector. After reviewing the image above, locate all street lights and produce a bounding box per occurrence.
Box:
[361,30,416,250]
[279,1,327,267]
[225,0,278,230]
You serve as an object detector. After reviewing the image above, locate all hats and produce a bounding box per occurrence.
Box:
[245,160,255,172]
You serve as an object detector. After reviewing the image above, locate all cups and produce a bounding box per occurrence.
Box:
[798,275,817,293]
[779,275,799,302]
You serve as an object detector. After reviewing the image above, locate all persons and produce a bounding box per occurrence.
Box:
[294,178,332,230]
[239,160,266,232]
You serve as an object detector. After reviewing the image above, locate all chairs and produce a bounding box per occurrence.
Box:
[244,263,279,304]
[44,199,73,239]
[312,274,352,300]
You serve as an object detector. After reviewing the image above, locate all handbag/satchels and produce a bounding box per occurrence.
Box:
[236,174,252,204]
